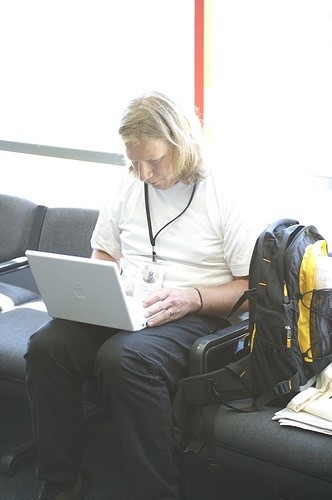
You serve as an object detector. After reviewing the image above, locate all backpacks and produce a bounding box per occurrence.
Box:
[245,219,332,406]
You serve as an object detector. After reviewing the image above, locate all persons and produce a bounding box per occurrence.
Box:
[23,91,259,500]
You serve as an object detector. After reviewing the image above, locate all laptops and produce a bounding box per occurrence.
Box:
[25,249,168,332]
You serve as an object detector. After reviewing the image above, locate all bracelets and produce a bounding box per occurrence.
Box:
[195,288,203,312]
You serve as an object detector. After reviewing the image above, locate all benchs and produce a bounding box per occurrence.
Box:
[0,194,332,500]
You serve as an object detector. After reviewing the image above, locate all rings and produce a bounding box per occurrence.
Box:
[167,309,173,315]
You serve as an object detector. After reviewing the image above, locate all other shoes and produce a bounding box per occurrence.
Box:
[39,464,90,500]
